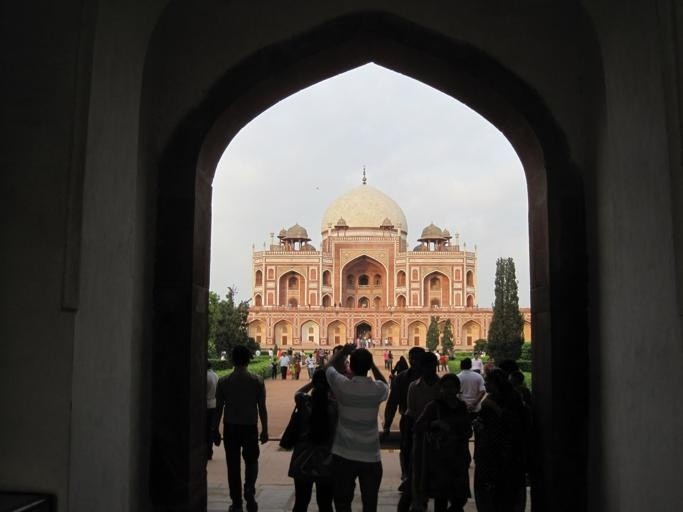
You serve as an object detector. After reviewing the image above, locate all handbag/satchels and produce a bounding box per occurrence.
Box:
[278,411,304,452]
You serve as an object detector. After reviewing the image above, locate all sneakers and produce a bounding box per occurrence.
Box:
[244,488,260,512]
[228,504,244,512]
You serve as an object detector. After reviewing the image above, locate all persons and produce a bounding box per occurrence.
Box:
[213,345,268,512]
[288,369,338,512]
[325,342,389,512]
[255,345,330,380]
[207,361,219,459]
[221,349,226,360]
[324,345,352,402]
[356,334,532,512]
